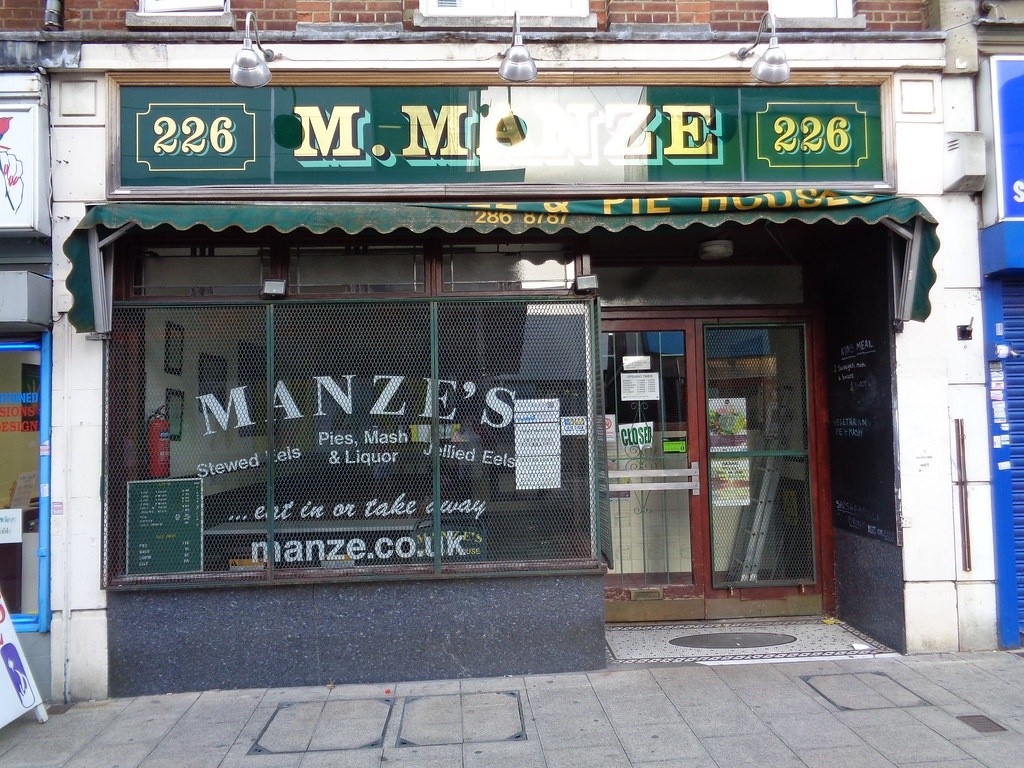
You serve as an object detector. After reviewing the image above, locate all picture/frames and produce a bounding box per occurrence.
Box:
[237,339,279,438]
[197,353,228,413]
[165,388,184,441]
[164,320,185,377]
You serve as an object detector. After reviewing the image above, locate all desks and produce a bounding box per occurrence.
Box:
[204,519,432,571]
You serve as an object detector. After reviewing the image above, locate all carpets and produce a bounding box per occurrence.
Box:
[605,616,896,665]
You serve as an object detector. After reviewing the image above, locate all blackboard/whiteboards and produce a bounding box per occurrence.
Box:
[125,477,206,579]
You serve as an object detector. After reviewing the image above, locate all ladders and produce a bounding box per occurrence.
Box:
[728,384,794,583]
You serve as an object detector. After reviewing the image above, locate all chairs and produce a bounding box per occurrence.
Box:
[410,517,488,563]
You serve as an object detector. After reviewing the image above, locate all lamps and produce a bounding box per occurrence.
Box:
[736,11,791,84]
[497,10,538,83]
[229,12,273,89]
[698,240,733,260]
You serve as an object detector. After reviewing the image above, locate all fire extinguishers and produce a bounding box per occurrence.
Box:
[145,403,171,476]
